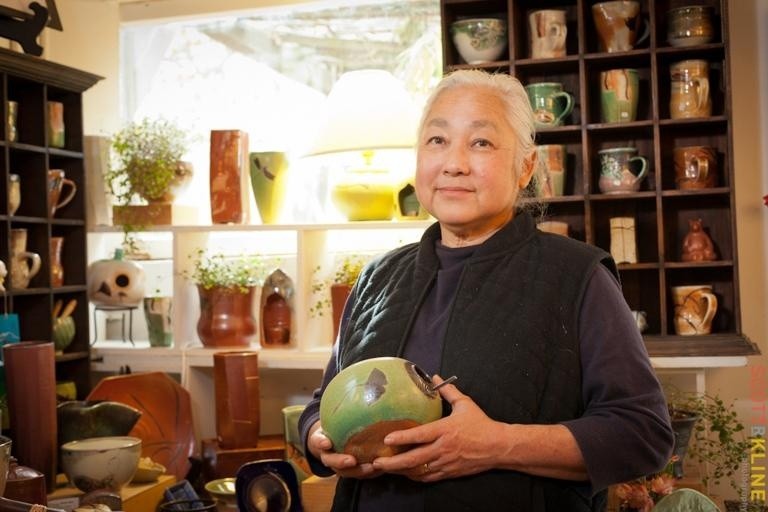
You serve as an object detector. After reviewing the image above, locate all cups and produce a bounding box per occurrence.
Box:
[143,294,173,348]
[7,170,77,290]
[450,0,718,337]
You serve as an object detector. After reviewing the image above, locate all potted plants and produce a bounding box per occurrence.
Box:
[650,378,768,512]
[180,248,261,349]
[101,118,192,258]
[308,252,368,347]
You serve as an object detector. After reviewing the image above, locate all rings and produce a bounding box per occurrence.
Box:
[423,463,429,474]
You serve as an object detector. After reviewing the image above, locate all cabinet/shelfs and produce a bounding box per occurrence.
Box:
[440,0,761,357]
[2,45,107,447]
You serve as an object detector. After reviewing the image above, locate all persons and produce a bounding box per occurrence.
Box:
[295,68,680,511]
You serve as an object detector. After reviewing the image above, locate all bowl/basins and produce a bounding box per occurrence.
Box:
[61,435,143,495]
